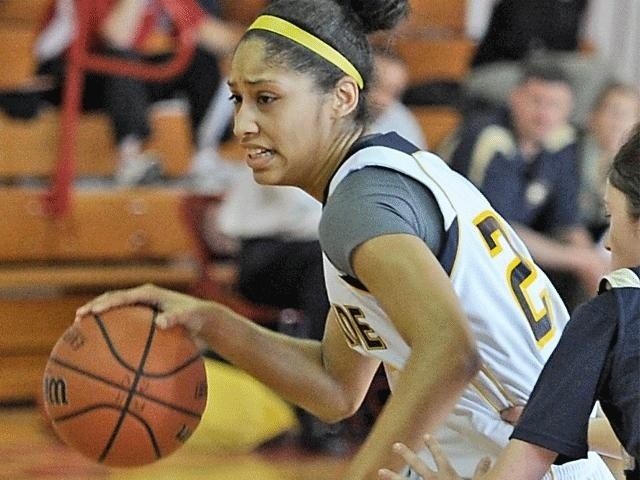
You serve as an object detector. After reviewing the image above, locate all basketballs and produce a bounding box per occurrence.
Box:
[43,305,208,467]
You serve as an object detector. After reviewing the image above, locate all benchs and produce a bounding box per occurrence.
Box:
[4,26,474,287]
